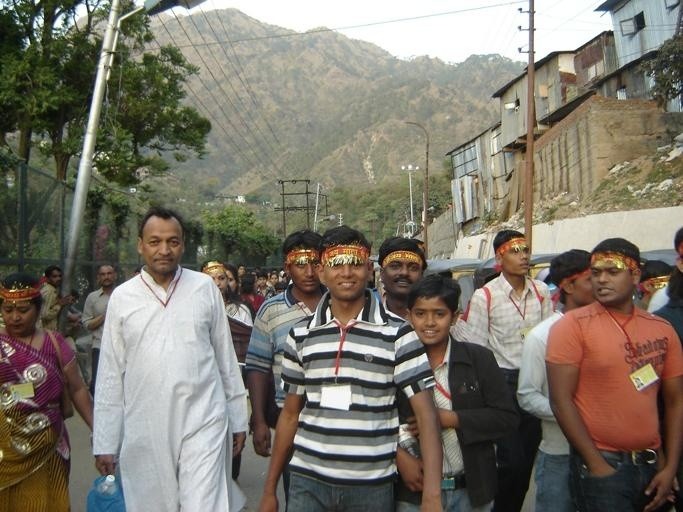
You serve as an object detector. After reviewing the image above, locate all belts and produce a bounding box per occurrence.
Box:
[440,476,465,490]
[597,448,659,462]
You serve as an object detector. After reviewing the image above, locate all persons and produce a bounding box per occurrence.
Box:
[200,258,254,481]
[513,247,590,512]
[38,265,75,333]
[88,204,251,512]
[257,225,444,511]
[1,272,93,512]
[634,225,683,346]
[243,228,324,511]
[543,236,682,512]
[393,272,520,512]
[58,287,80,336]
[466,228,553,512]
[82,264,118,394]
[409,238,425,257]
[377,233,474,343]
[225,262,294,316]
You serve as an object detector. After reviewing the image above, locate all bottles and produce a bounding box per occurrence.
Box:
[97,474,117,495]
[397,416,422,459]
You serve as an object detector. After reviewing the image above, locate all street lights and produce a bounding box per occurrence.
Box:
[314,215,337,233]
[400,164,420,233]
[60,1,209,300]
[400,120,430,260]
